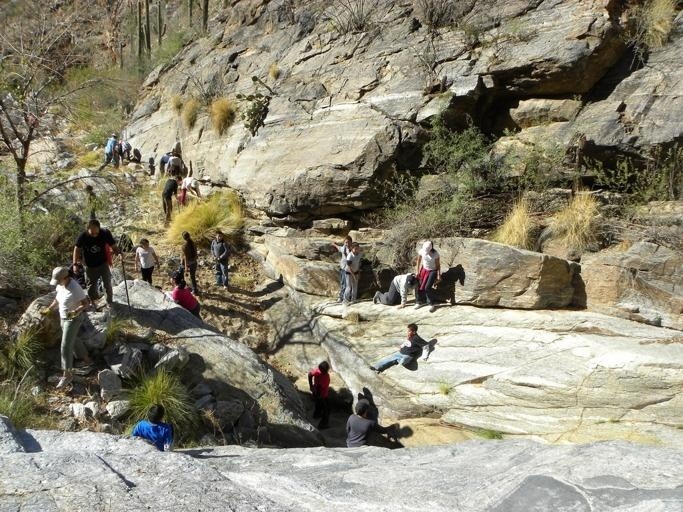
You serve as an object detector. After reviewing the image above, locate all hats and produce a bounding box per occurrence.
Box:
[422,241,432,254]
[50,266,69,285]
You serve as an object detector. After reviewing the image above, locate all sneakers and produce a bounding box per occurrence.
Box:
[77,362,97,375]
[414,302,436,313]
[55,373,74,388]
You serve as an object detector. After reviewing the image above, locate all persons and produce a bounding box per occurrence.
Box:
[370,323,423,370]
[415,239,440,312]
[131,404,173,452]
[71,126,230,318]
[326,236,351,302]
[371,273,418,309]
[343,242,364,305]
[345,400,399,447]
[40,266,95,389]
[307,359,334,432]
